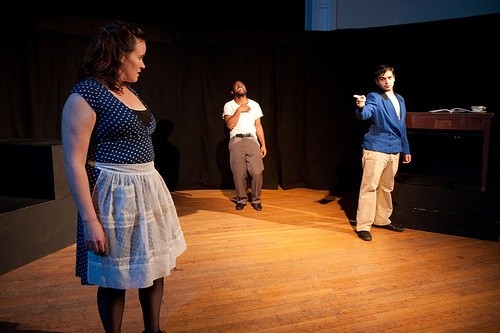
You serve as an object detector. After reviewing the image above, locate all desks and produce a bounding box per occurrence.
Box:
[406,112,495,191]
[0,138,73,201]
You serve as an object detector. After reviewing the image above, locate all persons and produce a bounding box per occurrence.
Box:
[353,64,411,242]
[61,16,187,333]
[222,80,267,211]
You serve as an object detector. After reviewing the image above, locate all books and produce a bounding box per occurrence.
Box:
[430,107,470,113]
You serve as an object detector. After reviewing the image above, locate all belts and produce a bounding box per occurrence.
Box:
[236,133,253,137]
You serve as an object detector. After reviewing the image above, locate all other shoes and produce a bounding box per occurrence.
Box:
[252,203,262,210]
[235,203,246,209]
[358,230,372,240]
[373,221,404,232]
